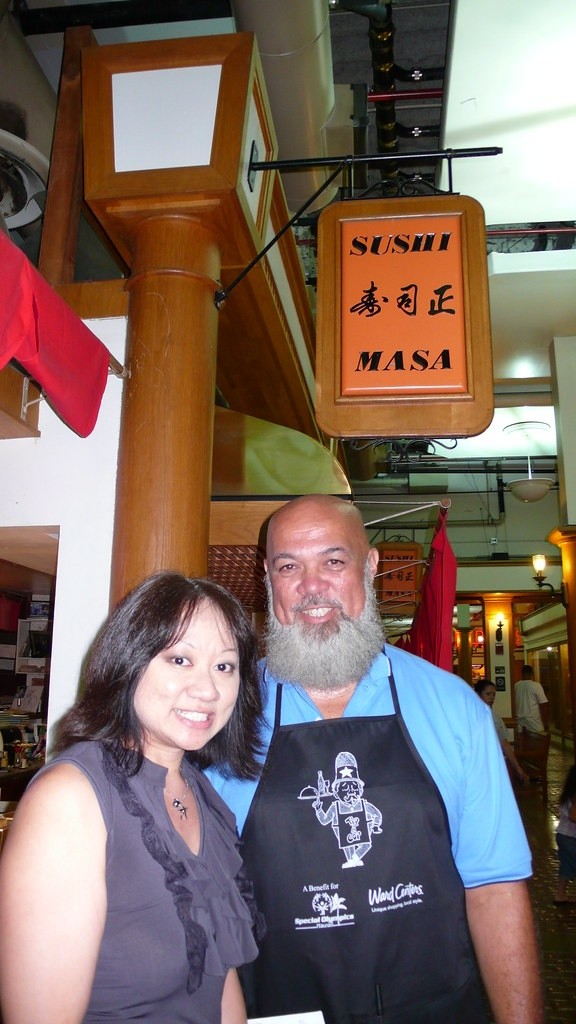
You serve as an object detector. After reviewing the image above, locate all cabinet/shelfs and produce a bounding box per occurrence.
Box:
[15,617,48,686]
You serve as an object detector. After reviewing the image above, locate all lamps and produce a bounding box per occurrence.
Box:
[503,422,555,503]
[495,612,504,642]
[531,554,569,609]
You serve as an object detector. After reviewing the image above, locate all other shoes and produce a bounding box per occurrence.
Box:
[530,776,543,784]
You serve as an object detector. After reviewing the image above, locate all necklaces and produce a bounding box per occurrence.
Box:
[110,746,189,822]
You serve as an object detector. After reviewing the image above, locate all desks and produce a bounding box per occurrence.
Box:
[0,760,45,801]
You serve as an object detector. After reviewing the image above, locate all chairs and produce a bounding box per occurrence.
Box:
[506,725,551,811]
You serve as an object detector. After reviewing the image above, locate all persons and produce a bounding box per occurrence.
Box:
[552,764,576,906]
[474,679,531,786]
[513,665,549,783]
[0,571,249,1024]
[200,493,546,1024]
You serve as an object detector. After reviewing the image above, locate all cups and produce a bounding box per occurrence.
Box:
[19,759,26,768]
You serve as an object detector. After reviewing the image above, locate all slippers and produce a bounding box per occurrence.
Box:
[553,896,576,905]
